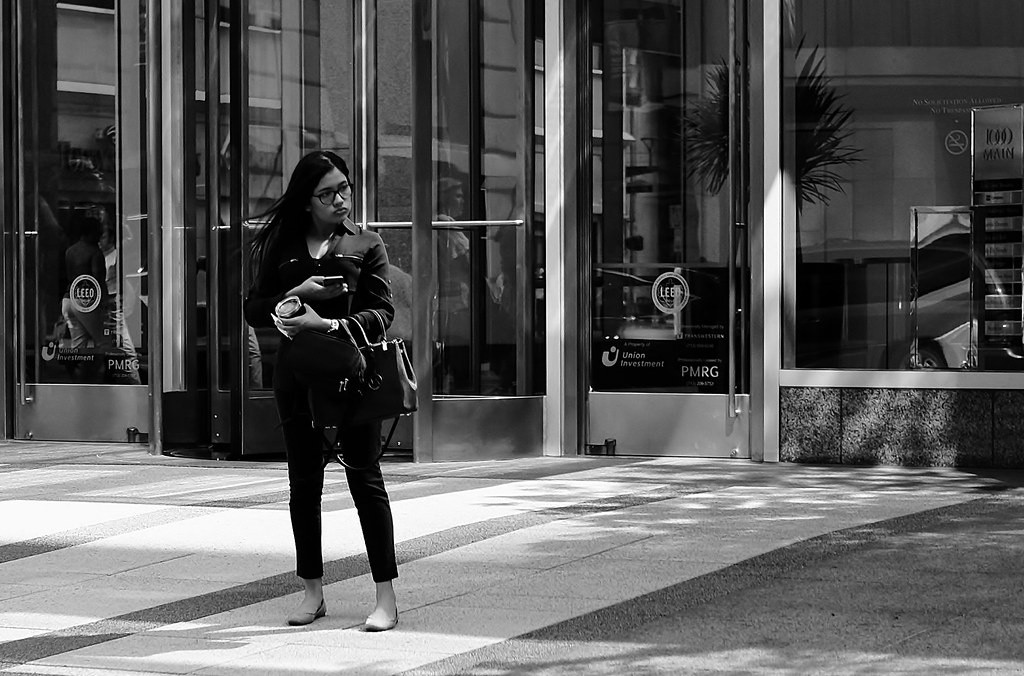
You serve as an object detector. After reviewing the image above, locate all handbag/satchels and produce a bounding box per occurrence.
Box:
[307,308,417,472]
[275,319,359,380]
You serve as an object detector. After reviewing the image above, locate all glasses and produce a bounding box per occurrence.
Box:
[312,182,354,207]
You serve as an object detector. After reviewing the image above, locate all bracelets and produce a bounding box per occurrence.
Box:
[327,319,339,332]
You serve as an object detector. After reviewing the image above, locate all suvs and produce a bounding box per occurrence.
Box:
[791,242,1015,373]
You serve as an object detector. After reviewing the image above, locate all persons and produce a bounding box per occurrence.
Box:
[244,152,398,632]
[61,217,111,347]
[432,178,469,313]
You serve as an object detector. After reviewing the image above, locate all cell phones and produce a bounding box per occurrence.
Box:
[323,276,344,287]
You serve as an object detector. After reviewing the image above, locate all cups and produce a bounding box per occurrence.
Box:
[274,296,305,318]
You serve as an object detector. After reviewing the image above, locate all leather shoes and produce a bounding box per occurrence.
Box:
[286,597,326,625]
[363,606,400,632]
